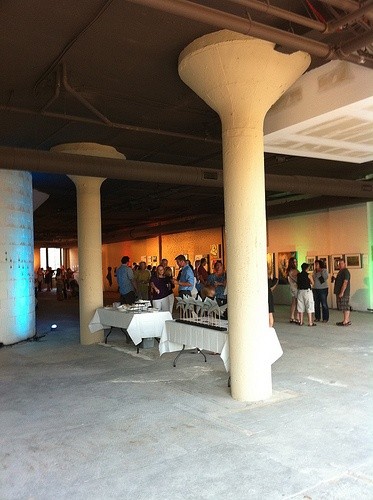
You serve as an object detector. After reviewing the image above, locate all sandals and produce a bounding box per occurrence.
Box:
[336,321,351,326]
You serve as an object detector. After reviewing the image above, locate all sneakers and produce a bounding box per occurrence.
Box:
[314,319,320,322]
[322,319,328,323]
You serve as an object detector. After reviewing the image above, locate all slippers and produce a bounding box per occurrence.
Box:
[308,323,316,326]
[299,323,304,326]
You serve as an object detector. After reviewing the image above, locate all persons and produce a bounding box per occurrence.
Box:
[201,286,222,356]
[37,254,228,320]
[311,259,329,323]
[295,263,317,326]
[286,257,300,324]
[330,259,351,326]
[267,261,279,327]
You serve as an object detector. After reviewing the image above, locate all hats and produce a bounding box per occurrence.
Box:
[301,263,311,270]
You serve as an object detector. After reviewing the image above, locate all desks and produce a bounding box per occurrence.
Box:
[158,317,283,387]
[88,305,173,354]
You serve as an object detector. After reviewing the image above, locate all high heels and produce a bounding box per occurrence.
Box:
[290,318,296,323]
[295,319,301,325]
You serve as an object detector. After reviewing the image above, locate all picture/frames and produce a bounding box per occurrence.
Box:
[305,254,361,273]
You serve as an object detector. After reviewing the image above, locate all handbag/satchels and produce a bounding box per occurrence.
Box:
[318,274,325,284]
